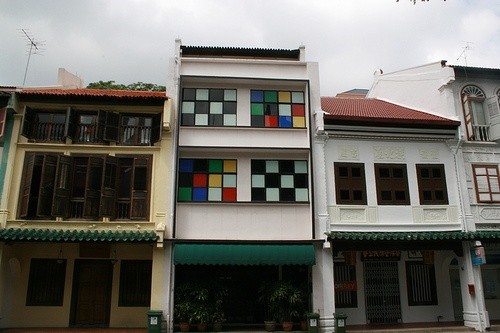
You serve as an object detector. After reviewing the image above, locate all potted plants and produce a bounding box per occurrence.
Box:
[250,284,280,331]
[269,283,304,331]
[187,287,218,330]
[298,289,309,329]
[210,289,226,331]
[174,293,199,331]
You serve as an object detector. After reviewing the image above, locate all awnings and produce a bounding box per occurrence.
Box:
[172,242,317,267]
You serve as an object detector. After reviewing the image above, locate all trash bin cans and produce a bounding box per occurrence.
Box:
[145,310,163,333]
[333,313,348,333]
[304,311,320,333]
